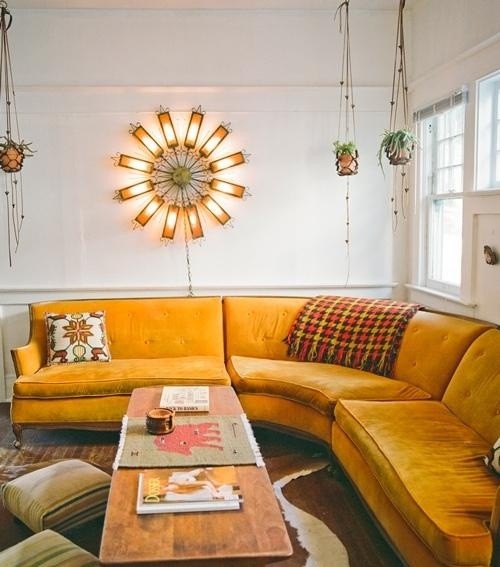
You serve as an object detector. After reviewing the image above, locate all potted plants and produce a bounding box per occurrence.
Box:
[331,139,361,292]
[377,126,419,233]
[0,136,37,267]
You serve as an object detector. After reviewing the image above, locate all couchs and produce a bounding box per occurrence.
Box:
[9,293,500,567]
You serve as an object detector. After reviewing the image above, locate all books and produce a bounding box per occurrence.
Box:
[159,386,209,412]
[136,466,243,514]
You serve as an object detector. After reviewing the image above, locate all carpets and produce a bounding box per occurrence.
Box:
[0,404,349,567]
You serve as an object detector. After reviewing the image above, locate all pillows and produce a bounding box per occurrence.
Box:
[484,436,500,475]
[0,457,113,535]
[41,310,111,365]
[0,529,101,566]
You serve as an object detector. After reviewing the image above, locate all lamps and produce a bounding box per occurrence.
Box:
[112,104,253,247]
